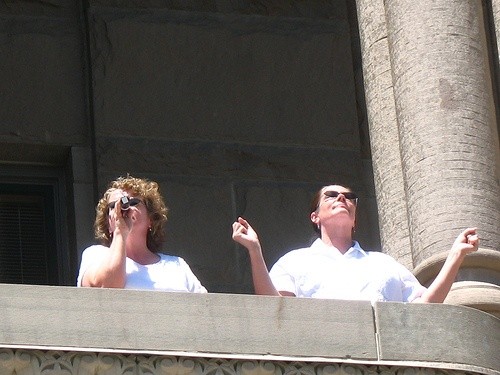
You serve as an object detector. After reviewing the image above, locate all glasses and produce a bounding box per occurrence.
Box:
[107,196,147,214]
[317,191,358,207]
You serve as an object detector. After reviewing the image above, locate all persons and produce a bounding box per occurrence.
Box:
[231,183,479,303]
[77,172,208,294]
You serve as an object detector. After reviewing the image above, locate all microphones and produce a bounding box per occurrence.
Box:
[121,197,131,217]
